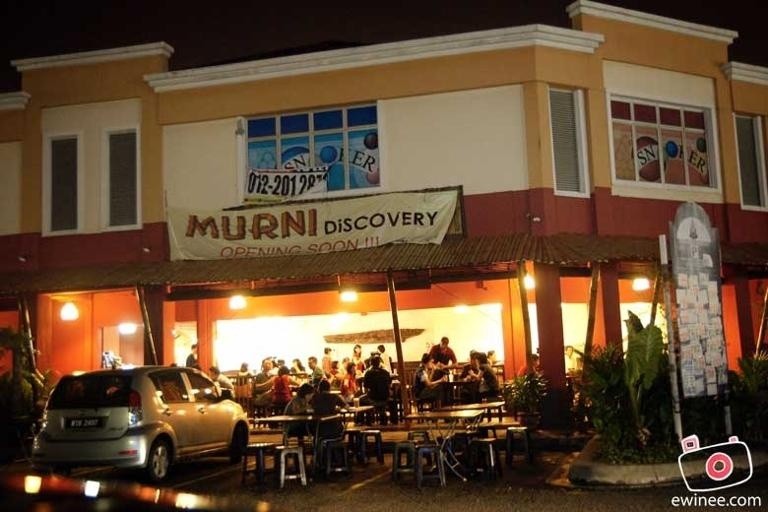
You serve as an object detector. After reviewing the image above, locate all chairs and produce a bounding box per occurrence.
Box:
[229,367,505,428]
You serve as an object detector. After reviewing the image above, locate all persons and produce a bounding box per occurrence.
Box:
[172,334,583,443]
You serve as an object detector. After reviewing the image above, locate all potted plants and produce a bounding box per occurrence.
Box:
[501,373,552,431]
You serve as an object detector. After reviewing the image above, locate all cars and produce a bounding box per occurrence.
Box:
[29,363,254,485]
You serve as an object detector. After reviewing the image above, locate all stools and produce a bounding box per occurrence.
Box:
[241,427,534,491]
